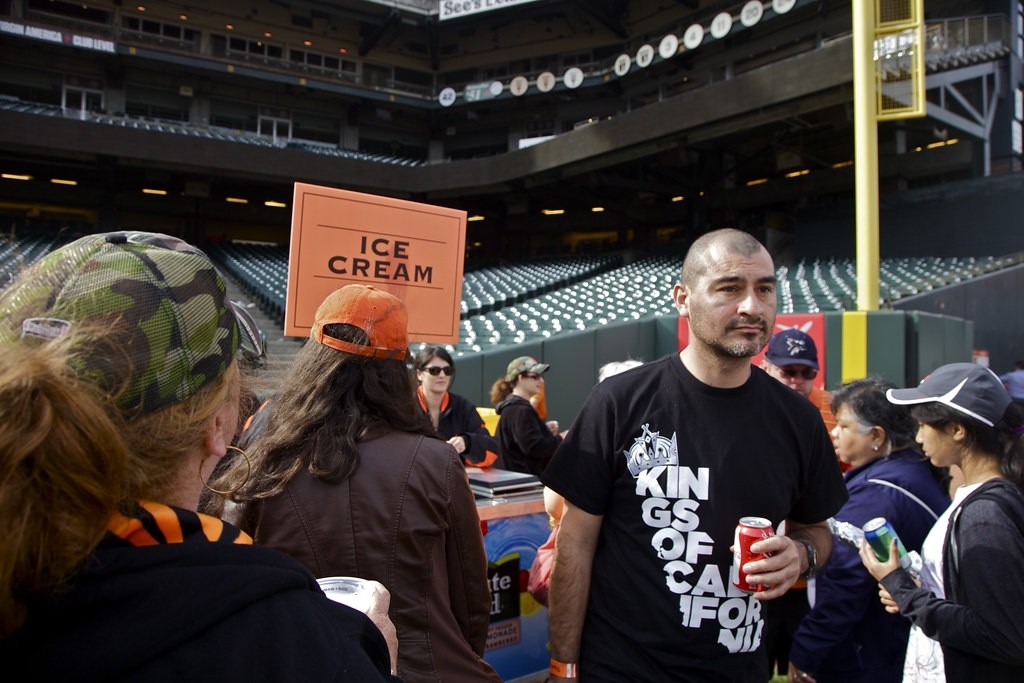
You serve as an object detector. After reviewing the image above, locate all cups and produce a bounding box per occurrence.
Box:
[315,577,375,616]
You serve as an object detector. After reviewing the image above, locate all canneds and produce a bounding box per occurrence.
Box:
[731,517,775,594]
[861,517,912,572]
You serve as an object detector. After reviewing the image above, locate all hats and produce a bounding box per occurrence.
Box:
[311,283,411,360]
[996,369,1024,399]
[2,229,270,424]
[884,361,1015,429]
[504,356,551,383]
[764,328,819,371]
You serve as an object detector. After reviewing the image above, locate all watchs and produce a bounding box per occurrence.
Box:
[798,541,819,580]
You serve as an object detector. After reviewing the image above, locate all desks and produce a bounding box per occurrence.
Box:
[468,494,556,683]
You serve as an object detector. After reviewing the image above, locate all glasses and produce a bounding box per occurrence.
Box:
[419,365,455,376]
[524,375,545,380]
[769,362,817,380]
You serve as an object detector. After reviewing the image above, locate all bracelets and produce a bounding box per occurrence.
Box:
[550,659,578,678]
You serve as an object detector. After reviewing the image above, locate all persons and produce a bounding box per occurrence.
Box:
[859,363,1024,683]
[763,328,819,396]
[547,229,850,683]
[492,356,566,477]
[412,346,498,464]
[208,284,502,683]
[543,361,643,520]
[0,232,402,683]
[788,377,951,683]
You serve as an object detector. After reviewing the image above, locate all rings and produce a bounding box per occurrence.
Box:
[793,674,799,679]
[800,673,807,677]
